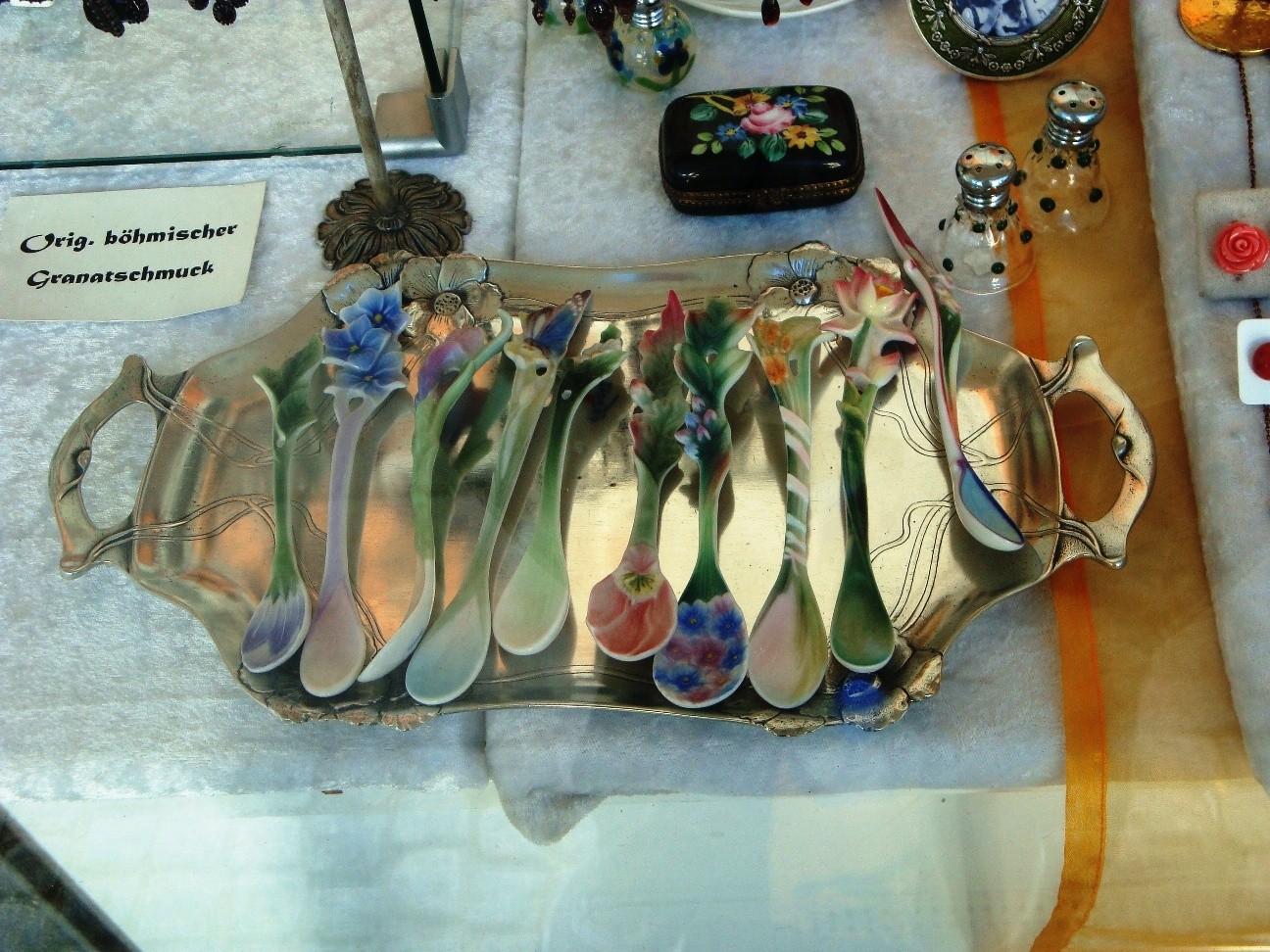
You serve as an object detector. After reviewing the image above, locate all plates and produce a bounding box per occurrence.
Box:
[1194,183,1270,407]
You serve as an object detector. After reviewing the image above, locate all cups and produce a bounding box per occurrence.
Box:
[241,184,1030,717]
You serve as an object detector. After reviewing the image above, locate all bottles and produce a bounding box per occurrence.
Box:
[1021,80,1111,236]
[929,140,1037,296]
